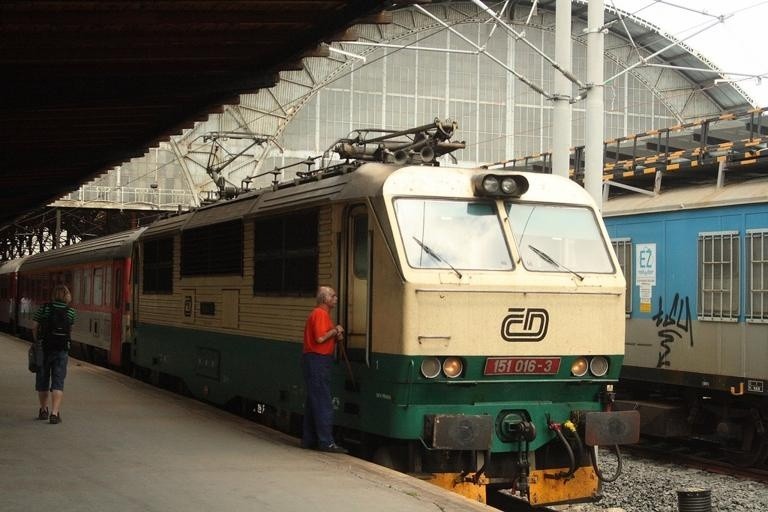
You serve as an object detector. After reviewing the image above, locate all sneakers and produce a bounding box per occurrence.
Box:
[37,406,62,426]
[315,438,350,455]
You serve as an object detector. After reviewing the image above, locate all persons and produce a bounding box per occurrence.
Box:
[31,284,76,424]
[300,287,347,453]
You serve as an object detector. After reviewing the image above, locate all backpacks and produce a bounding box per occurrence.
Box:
[43,300,71,349]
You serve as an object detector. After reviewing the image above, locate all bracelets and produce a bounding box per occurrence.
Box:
[335,328,339,334]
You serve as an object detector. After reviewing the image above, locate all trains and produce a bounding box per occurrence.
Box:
[1,112,640,512]
[597,172,766,482]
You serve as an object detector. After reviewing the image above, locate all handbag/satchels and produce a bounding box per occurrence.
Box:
[29,338,48,375]
